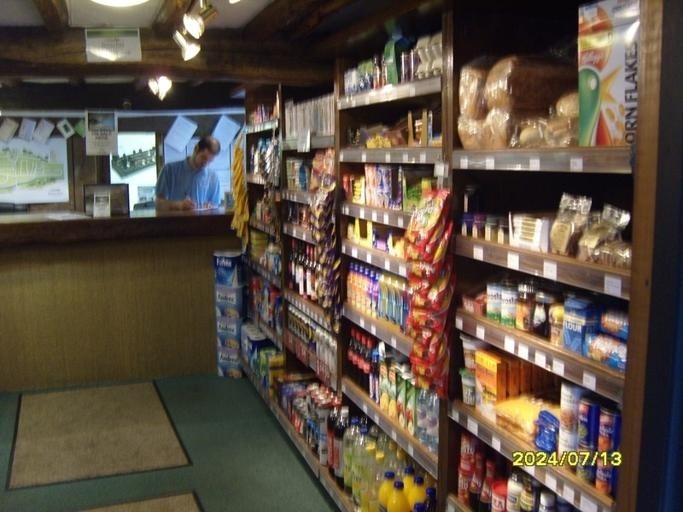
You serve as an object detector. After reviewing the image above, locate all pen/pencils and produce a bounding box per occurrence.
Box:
[184,192,190,201]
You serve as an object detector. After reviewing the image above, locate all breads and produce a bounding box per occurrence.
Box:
[457,53,580,149]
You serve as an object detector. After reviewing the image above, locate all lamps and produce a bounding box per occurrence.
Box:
[170,0,218,63]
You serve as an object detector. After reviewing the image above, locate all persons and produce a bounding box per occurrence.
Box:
[152,137,221,211]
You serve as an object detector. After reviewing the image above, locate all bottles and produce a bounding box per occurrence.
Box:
[513,282,553,339]
[341,327,375,395]
[469,454,573,512]
[282,236,320,302]
[328,396,438,512]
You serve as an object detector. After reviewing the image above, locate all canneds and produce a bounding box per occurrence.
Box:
[485,277,557,340]
[557,380,622,501]
[400,51,419,84]
